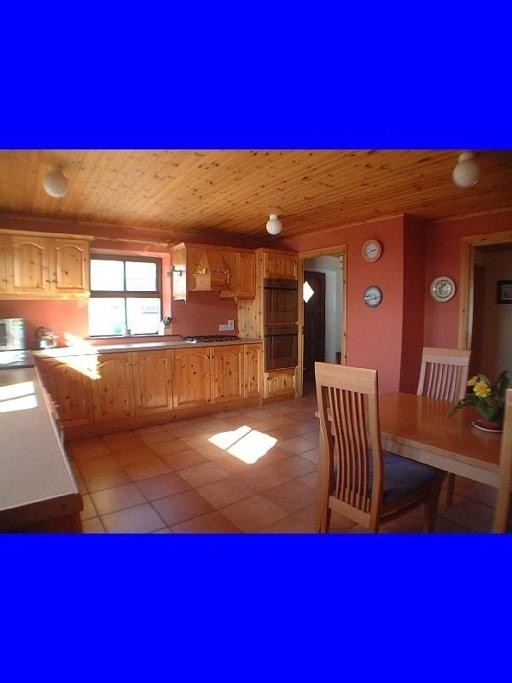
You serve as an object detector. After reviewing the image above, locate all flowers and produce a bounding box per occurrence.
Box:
[449,370,512,424]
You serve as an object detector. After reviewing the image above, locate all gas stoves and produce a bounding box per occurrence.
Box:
[186,333,240,343]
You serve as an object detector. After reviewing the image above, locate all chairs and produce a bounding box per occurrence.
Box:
[315,361,449,534]
[488,387,512,533]
[416,347,471,507]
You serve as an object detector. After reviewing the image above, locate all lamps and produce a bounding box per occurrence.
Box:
[41,166,69,197]
[266,214,282,235]
[452,151,480,188]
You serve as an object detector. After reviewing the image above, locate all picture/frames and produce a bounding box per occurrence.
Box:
[496,280,512,303]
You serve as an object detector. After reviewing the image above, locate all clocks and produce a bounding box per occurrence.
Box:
[431,276,456,302]
[363,286,383,307]
[362,239,382,262]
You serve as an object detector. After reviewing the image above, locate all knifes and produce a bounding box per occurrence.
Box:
[162,315,173,326]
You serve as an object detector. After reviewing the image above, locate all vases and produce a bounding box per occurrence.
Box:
[478,414,503,430]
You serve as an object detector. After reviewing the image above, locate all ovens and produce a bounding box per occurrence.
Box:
[0,316,27,351]
[264,276,299,371]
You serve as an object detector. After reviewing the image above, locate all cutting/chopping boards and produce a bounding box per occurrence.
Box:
[0,350,34,370]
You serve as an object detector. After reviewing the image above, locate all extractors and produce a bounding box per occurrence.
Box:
[188,269,234,291]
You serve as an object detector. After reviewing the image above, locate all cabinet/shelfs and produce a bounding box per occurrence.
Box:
[172,344,244,424]
[243,343,264,409]
[15,512,84,533]
[171,241,256,303]
[91,350,174,440]
[237,248,300,406]
[36,354,94,443]
[0,228,95,301]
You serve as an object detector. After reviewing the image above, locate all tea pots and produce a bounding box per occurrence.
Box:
[33,326,59,349]
